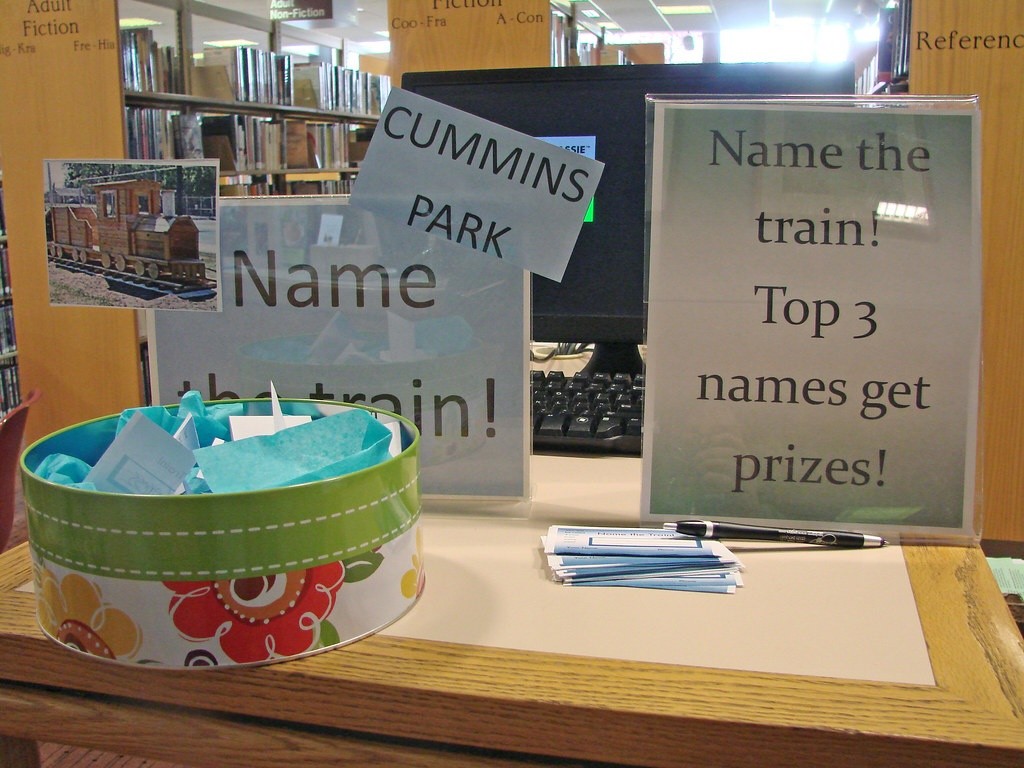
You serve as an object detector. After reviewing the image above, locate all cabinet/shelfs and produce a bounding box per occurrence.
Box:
[1,0,1023,768]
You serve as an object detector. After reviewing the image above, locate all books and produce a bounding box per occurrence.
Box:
[0,211,26,423]
[122,24,390,196]
[549,13,638,68]
[854,0,912,100]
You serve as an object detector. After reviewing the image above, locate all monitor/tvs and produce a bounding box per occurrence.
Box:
[401,61,856,380]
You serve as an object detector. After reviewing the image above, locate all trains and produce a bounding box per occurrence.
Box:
[48,179,207,282]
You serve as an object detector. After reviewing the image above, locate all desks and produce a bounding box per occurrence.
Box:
[3,341,1022,768]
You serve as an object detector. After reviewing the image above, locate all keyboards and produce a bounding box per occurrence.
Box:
[528,369,645,459]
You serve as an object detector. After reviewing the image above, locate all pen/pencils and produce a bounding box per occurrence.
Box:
[661,518,891,550]
